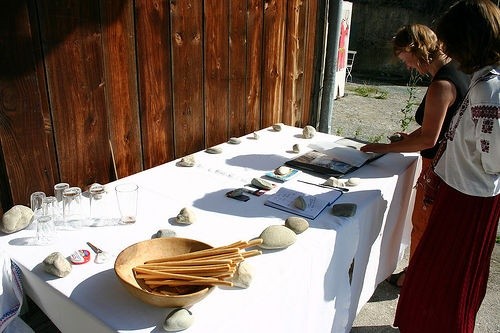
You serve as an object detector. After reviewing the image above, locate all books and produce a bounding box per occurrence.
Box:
[286,135,388,182]
[263,178,344,220]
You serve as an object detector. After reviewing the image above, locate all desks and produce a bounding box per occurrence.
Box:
[0,123,423,333]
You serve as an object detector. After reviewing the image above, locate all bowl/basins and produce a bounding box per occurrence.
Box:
[114,237,217,309]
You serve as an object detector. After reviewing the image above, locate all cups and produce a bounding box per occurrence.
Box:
[115,183,139,223]
[30,181,110,245]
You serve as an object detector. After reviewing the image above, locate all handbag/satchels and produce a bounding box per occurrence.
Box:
[421,167,442,206]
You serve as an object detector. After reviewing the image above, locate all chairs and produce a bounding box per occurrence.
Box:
[345,51,357,83]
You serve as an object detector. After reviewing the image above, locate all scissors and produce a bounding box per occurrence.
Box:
[87,242,111,263]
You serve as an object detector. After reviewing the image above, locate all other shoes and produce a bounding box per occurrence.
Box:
[385,270,407,288]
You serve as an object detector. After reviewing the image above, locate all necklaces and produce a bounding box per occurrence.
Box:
[446,64,492,139]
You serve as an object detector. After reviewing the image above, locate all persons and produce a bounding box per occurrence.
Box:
[359,25,470,288]
[393,0,500,333]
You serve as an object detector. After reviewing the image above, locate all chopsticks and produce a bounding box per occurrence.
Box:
[132,237,264,288]
